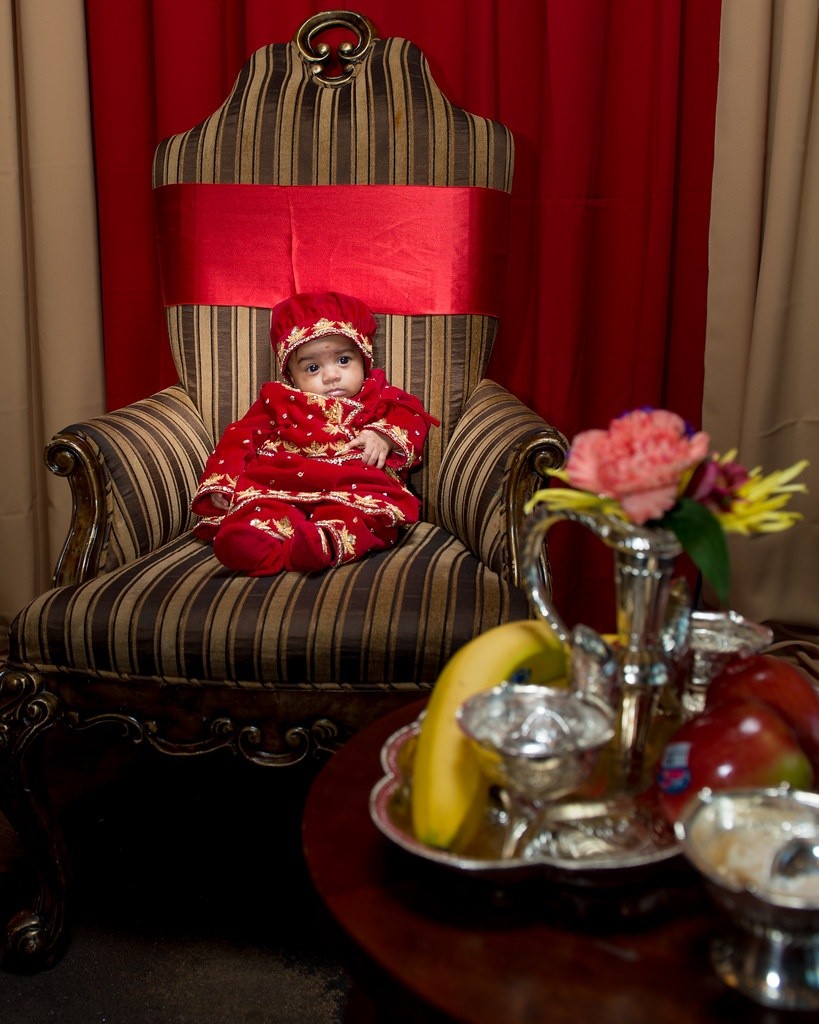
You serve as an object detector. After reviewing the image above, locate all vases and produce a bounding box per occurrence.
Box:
[614,528,700,797]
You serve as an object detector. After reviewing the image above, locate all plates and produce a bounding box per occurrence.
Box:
[369,710,684,888]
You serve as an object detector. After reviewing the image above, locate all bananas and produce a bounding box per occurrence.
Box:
[408,616,592,855]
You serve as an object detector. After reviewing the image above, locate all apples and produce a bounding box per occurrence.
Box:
[657,652,819,832]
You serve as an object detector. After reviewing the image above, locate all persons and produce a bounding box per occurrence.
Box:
[189,291,440,577]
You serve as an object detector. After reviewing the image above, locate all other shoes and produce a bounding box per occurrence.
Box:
[285,521,332,572]
[215,523,285,574]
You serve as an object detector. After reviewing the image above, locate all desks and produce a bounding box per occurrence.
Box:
[302,695,747,1024]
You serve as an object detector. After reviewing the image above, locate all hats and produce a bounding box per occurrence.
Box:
[271,291,376,382]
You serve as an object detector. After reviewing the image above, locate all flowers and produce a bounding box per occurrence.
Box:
[527,408,812,611]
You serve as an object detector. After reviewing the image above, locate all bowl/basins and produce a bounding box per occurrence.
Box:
[687,610,773,686]
[459,684,615,800]
[674,787,819,933]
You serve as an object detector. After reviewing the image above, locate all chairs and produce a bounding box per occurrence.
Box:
[0,10,573,973]
